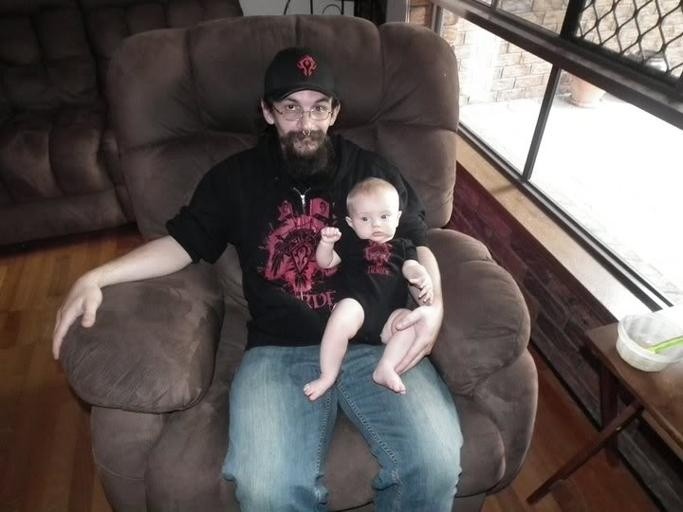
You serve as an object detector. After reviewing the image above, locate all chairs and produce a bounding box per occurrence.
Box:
[57,14,539,512]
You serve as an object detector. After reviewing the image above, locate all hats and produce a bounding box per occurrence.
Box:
[264,46,338,105]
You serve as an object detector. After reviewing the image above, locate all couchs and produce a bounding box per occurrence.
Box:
[1,1,244,252]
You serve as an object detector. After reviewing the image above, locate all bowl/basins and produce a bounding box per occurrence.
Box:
[613,312,683,373]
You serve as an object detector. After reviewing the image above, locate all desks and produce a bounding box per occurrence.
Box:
[527,303,683,511]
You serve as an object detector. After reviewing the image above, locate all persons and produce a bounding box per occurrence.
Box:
[303,176,435,402]
[50,46,465,512]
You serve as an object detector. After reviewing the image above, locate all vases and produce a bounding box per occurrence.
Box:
[568,73,606,109]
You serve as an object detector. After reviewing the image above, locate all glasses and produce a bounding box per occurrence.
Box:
[272,104,333,123]
